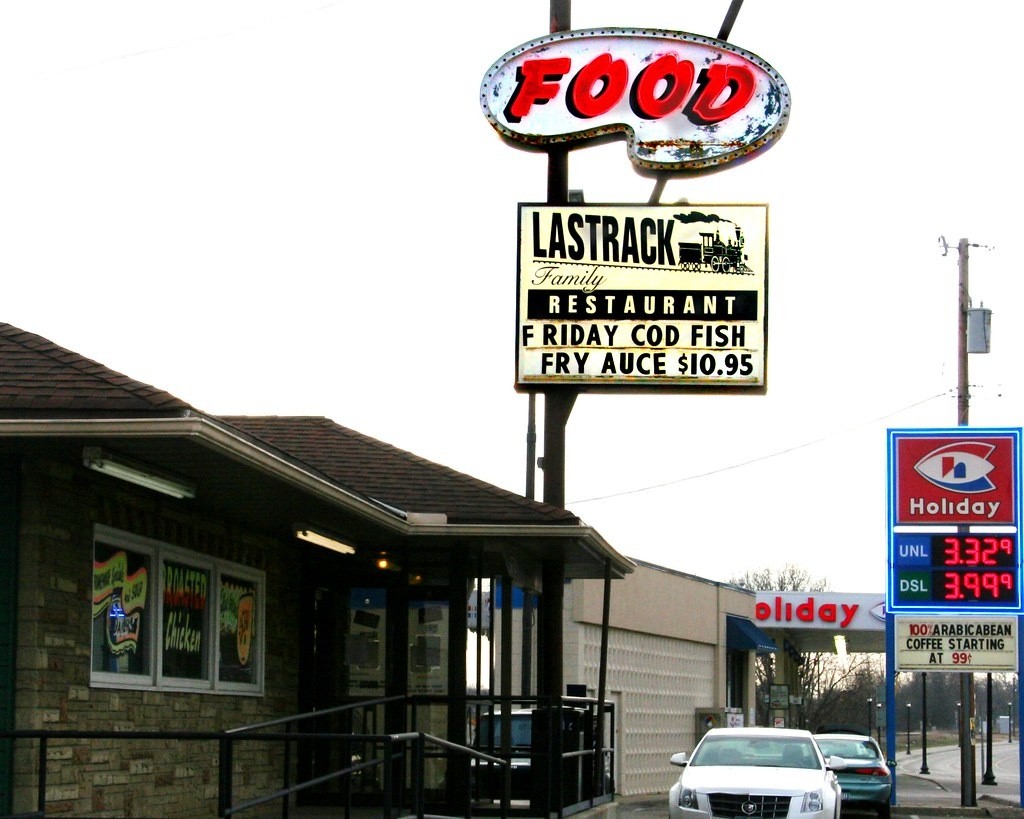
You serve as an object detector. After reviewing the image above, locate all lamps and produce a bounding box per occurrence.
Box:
[81,445,196,500]
[293,521,359,556]
[376,549,390,568]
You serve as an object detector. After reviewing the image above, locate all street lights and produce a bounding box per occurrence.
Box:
[905,699,912,754]
[875,700,882,744]
[955,700,961,747]
[1007,702,1012,744]
[866,693,874,736]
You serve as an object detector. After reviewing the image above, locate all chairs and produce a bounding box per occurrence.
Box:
[716,748,742,764]
[778,745,806,767]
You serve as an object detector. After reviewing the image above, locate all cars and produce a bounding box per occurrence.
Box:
[668,727,849,819]
[810,733,893,819]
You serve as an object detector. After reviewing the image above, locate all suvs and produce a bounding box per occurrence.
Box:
[463,704,606,806]
